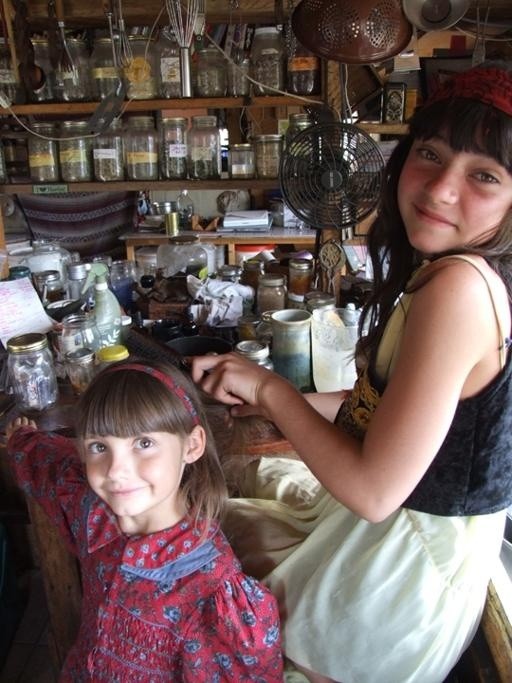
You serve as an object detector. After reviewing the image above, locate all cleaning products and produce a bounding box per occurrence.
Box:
[80,262,120,337]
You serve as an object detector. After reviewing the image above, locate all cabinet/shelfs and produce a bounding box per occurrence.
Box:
[0,0,512,323]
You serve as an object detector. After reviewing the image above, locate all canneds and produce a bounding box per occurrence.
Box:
[288,259,313,294]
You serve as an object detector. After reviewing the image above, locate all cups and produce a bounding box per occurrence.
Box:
[310,308,370,393]
[270,306,310,393]
[165,213,180,236]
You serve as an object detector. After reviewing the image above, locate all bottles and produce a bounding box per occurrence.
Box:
[176,189,194,226]
[7,235,208,418]
[0,25,318,183]
[217,258,337,372]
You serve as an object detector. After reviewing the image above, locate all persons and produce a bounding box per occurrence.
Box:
[187,58,512,683]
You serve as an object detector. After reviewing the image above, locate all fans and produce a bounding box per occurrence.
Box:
[279,122,386,230]
